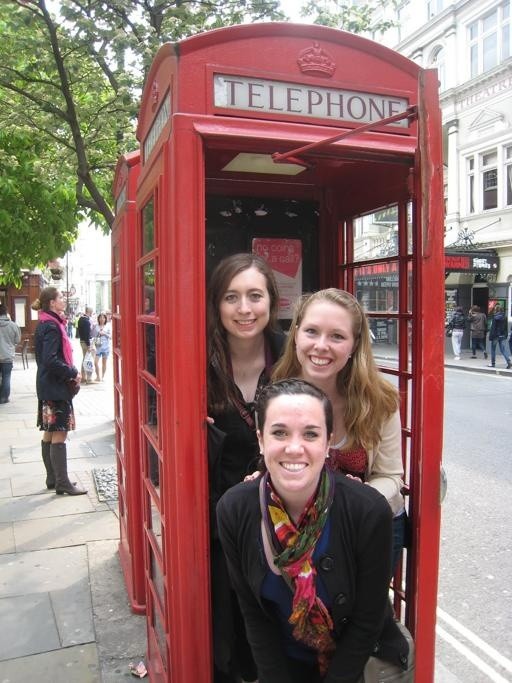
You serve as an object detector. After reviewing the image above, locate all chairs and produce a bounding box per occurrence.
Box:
[13,338,30,370]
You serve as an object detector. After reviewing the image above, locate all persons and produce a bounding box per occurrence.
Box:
[269,287,407,584]
[216,378,415,683]
[0,305,21,404]
[467,306,488,359]
[446,306,466,360]
[32,287,88,494]
[206,253,291,683]
[65,305,112,384]
[486,305,512,369]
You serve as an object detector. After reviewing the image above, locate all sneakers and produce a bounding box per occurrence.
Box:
[453,351,512,369]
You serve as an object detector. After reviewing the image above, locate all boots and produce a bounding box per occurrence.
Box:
[40,439,88,497]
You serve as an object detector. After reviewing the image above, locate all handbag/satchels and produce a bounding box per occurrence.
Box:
[445,328,453,338]
[93,331,103,345]
[64,377,80,397]
